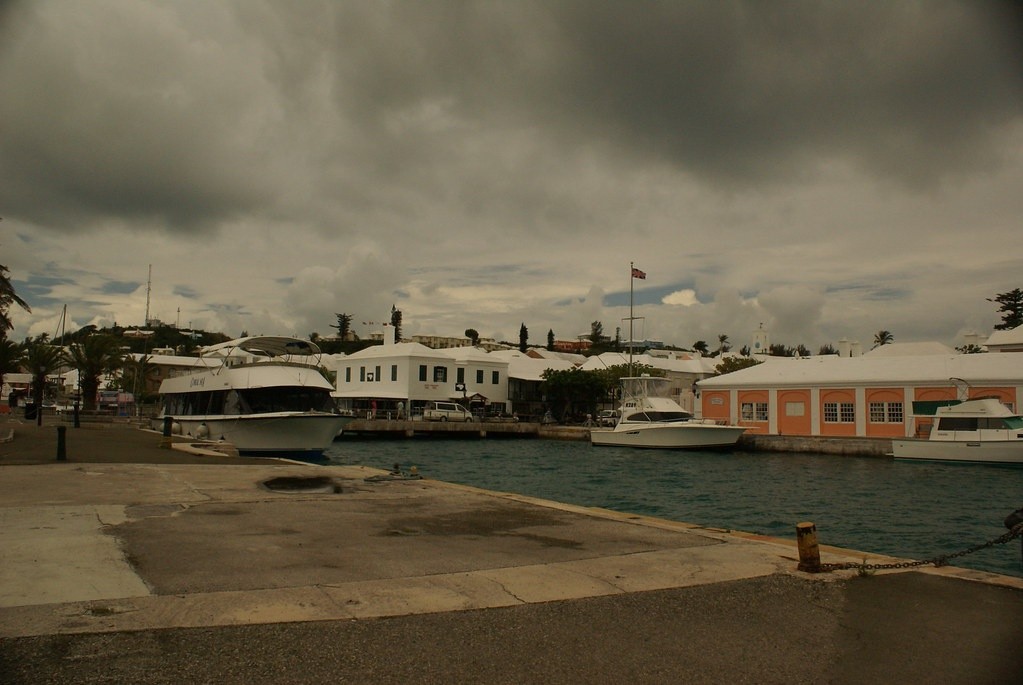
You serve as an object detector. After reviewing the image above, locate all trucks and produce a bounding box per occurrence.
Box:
[596,410,620,426]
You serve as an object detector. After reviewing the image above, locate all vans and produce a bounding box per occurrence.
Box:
[423,401,473,423]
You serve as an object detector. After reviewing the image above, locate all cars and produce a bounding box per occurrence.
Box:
[99,391,119,408]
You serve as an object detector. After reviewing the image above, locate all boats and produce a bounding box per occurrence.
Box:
[590,372,761,452]
[152,335,356,456]
[890,397,1023,467]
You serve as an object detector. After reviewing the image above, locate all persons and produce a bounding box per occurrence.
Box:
[371,399,376,419]
[396,400,404,422]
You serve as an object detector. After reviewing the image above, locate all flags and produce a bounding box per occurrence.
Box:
[632,268,646,280]
